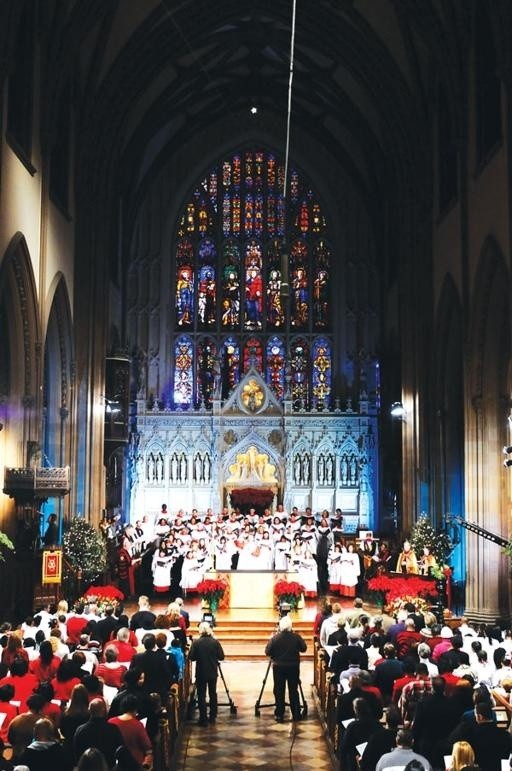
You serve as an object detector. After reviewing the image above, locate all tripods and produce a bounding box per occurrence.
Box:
[186,646,239,718]
[254,631,310,716]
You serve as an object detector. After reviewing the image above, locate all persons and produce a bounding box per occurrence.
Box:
[364,531,436,577]
[127,501,360,598]
[265,616,310,721]
[191,622,224,723]
[1,594,189,770]
[312,599,512,770]
[43,514,59,546]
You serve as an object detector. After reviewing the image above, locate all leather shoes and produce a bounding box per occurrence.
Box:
[273,714,284,723]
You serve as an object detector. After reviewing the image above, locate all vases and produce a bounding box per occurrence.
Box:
[208,596,218,613]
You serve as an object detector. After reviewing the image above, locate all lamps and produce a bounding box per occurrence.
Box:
[502,446,512,468]
[389,401,407,424]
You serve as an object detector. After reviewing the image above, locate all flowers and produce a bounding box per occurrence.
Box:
[273,581,304,603]
[367,576,439,605]
[196,579,227,600]
[84,585,125,610]
